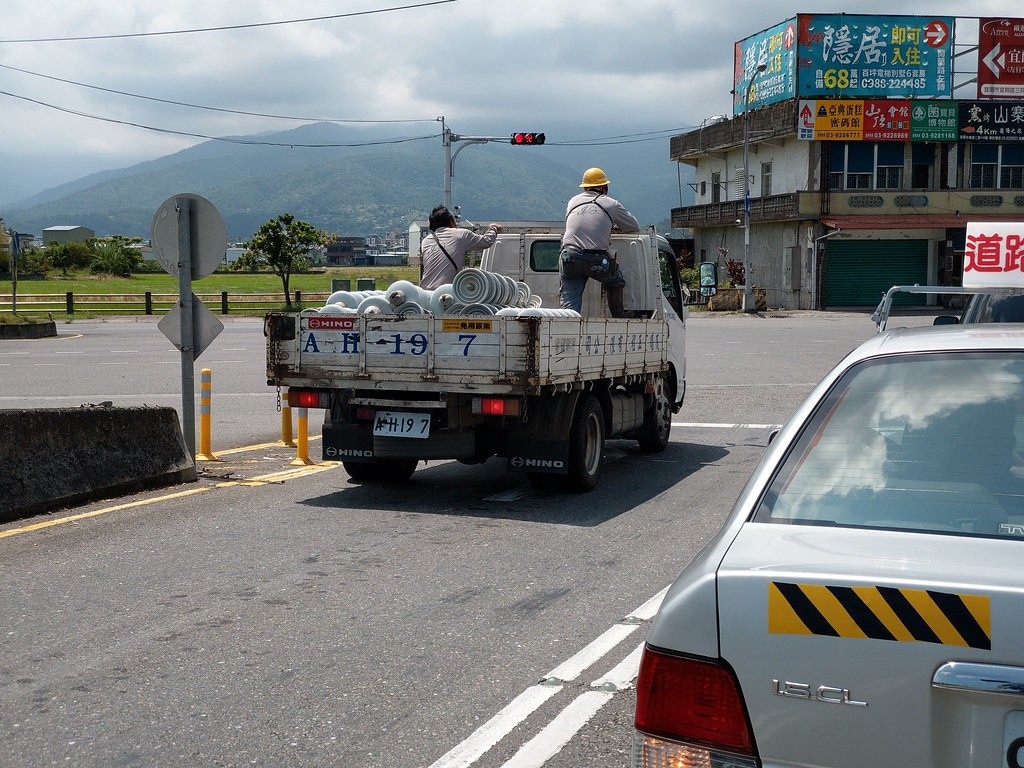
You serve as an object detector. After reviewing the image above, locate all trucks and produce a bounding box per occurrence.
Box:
[263,225,719,496]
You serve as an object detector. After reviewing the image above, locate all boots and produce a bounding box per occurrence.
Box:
[606,283,634,318]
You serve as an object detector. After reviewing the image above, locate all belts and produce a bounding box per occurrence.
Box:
[568,245,607,254]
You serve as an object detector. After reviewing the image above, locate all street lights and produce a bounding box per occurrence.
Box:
[741,63,769,313]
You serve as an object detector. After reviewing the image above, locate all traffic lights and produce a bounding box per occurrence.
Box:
[513,132,546,146]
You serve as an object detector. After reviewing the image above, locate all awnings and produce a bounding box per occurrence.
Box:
[820,217,1024,229]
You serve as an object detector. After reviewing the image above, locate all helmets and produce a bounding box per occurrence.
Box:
[578,168,610,187]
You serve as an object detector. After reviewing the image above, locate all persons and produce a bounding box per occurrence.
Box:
[557,166,639,319]
[420,204,503,292]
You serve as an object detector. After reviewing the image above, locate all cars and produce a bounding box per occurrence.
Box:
[930,289,1024,328]
[630,321,1024,768]
